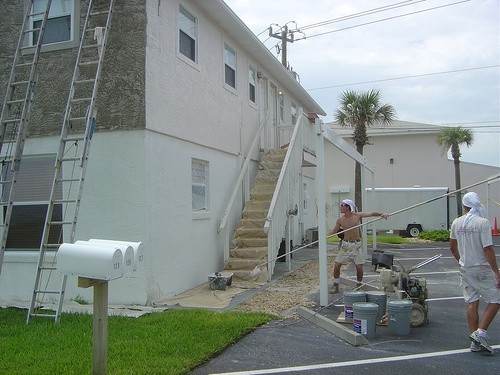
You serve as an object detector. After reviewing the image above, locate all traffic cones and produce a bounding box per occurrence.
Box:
[492,217,500,236]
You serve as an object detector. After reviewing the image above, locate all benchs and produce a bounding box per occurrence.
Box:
[207,271,234,291]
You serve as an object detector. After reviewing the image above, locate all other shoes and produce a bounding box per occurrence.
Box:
[356,283,364,291]
[330,282,338,293]
[469,328,493,352]
[470,341,485,351]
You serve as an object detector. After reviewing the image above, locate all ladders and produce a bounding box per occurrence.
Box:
[25,0,115,326]
[0,0,50,276]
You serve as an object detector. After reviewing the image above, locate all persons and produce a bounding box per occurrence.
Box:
[449,191,500,356]
[327,199,390,294]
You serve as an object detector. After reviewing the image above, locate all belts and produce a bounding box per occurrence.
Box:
[344,240,360,243]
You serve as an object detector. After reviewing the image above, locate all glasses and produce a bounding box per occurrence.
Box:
[341,205,345,206]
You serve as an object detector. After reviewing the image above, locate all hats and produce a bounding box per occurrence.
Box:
[342,199,356,212]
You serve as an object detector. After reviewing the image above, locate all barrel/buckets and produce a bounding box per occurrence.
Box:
[352,302,379,338]
[366,291,387,318]
[385,289,412,336]
[343,290,367,324]
